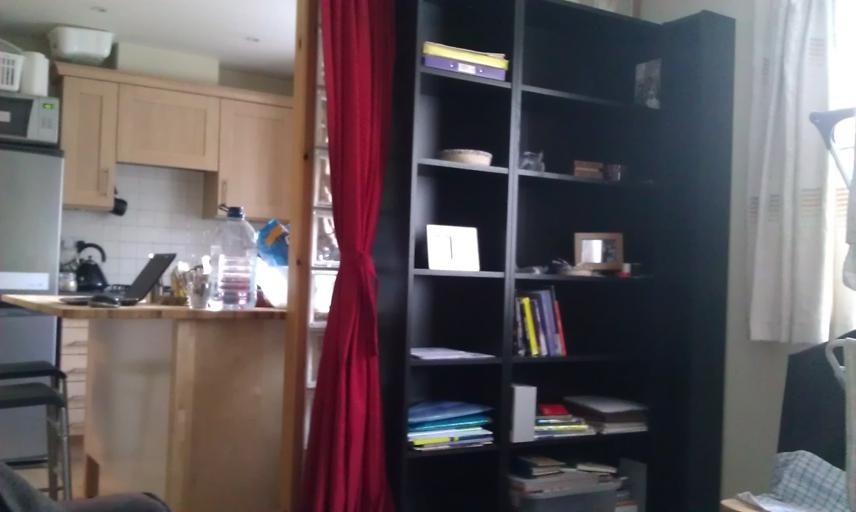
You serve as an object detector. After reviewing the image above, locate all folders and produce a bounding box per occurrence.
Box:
[420,42,510,82]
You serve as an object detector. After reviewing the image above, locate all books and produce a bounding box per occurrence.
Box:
[517,456,566,478]
[534,394,650,437]
[578,462,638,512]
[421,41,510,81]
[407,426,493,452]
[514,284,568,359]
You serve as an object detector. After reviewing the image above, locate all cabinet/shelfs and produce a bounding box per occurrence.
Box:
[373,0,738,512]
[49,61,296,224]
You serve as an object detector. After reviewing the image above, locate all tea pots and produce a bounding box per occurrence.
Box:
[70,243,109,293]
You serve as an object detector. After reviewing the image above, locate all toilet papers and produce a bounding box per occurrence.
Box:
[19,49,50,97]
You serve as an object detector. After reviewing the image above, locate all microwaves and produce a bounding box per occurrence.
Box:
[0,84,61,149]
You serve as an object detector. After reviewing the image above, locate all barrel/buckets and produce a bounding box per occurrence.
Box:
[212,198,258,310]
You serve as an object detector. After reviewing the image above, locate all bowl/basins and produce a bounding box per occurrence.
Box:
[440,149,494,167]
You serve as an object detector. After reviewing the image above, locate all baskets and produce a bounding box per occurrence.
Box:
[0,51,24,91]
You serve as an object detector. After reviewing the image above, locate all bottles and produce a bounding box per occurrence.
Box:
[59,272,78,294]
[210,205,258,314]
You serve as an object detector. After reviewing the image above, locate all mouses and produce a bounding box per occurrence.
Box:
[88,292,122,309]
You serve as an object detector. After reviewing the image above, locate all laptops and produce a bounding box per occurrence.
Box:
[59,252,177,304]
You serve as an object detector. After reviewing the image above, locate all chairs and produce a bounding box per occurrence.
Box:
[0,460,171,512]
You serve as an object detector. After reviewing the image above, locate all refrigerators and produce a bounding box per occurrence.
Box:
[1,141,69,468]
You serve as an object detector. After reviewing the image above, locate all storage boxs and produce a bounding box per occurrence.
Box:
[110,44,219,83]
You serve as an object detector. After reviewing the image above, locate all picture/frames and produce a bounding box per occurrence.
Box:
[573,231,625,272]
